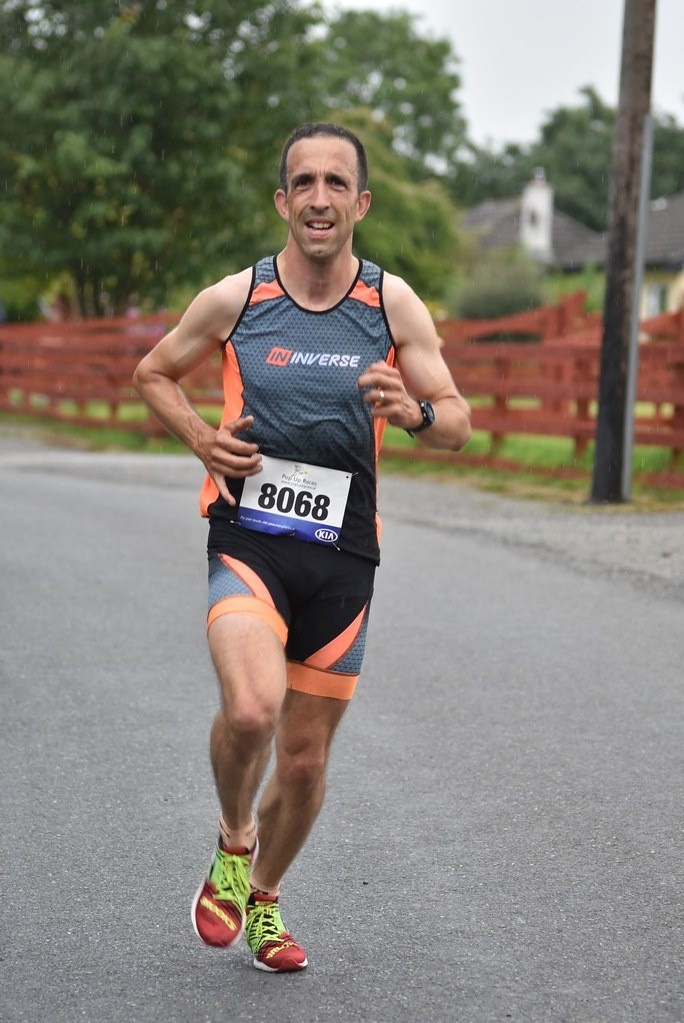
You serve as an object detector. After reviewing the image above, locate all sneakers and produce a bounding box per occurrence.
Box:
[245,894,309,972]
[188,836,261,952]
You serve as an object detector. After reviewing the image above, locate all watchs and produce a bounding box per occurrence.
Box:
[403,397,433,438]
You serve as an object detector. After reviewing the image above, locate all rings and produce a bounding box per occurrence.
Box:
[378,391,385,404]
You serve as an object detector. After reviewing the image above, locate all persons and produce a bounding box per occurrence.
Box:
[133,125,473,971]
